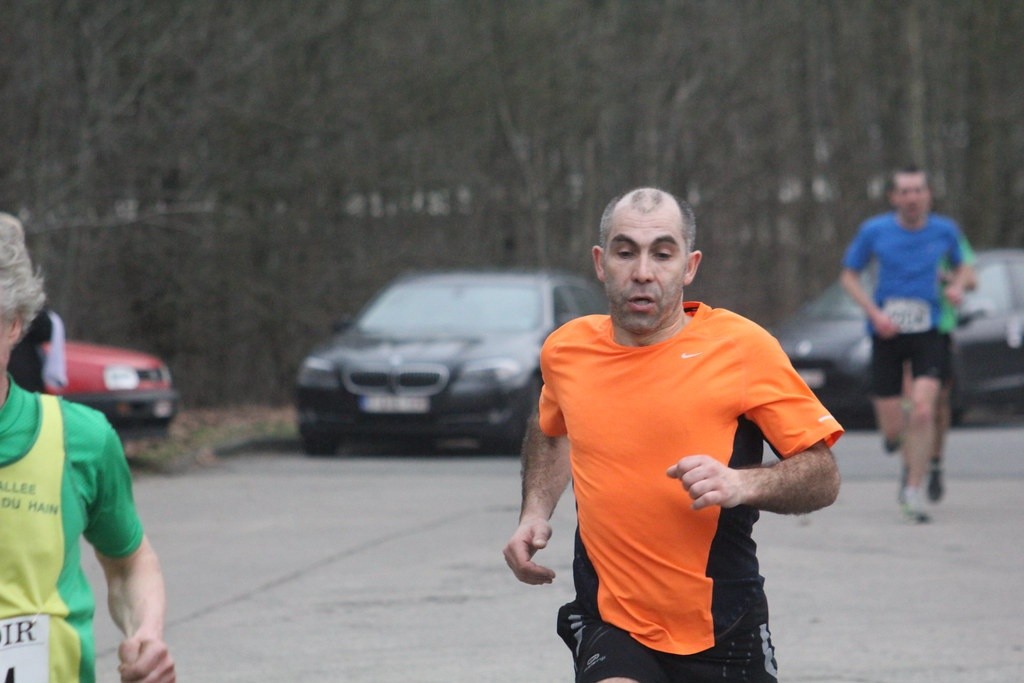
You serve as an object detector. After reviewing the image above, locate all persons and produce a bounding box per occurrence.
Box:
[839,162,982,528]
[502,186,846,683]
[0,211,178,683]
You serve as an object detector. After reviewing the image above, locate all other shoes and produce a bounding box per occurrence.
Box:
[926,462,942,501]
[898,487,929,520]
[884,435,901,452]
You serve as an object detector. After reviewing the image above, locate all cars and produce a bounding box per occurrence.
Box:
[293,264,610,455]
[775,245,1024,435]
[39,336,183,424]
